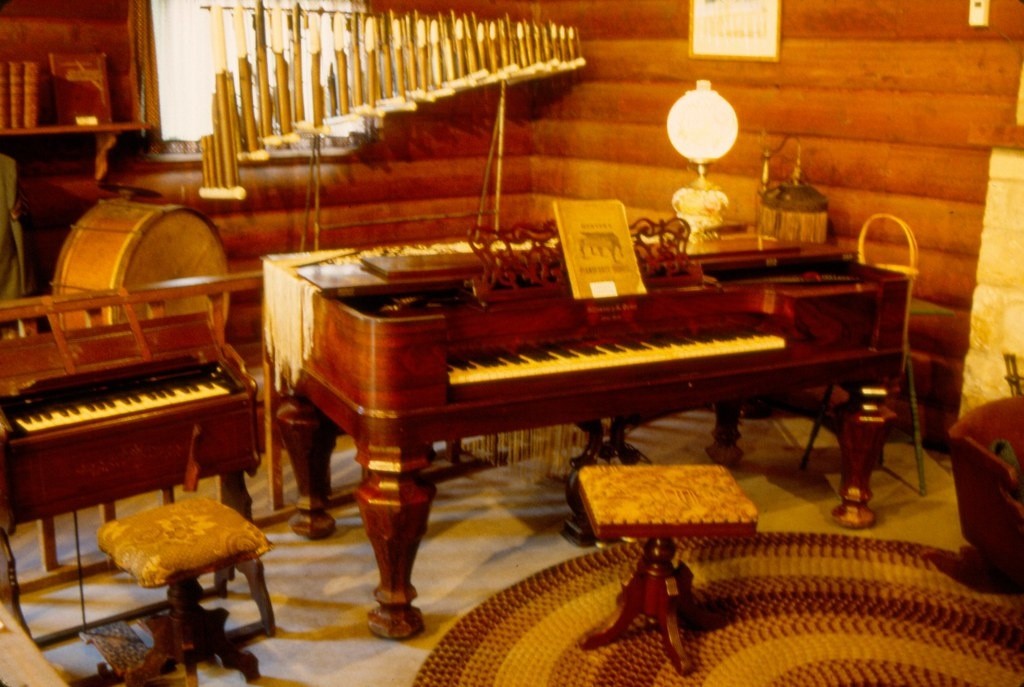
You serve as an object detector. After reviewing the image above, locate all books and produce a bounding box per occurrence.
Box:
[22,61,39,127]
[551,195,646,301]
[8,61,24,128]
[0,59,9,129]
[49,51,112,125]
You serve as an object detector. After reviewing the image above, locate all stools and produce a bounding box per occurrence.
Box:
[97,495,269,687]
[577,463,758,675]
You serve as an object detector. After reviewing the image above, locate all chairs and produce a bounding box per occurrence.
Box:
[948,395,1024,592]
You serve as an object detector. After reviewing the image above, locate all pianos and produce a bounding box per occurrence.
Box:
[0,310,278,687]
[258,218,916,643]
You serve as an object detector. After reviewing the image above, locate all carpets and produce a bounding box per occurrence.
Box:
[411,533,1024,687]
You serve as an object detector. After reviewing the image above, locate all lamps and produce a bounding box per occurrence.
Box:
[668,77,739,229]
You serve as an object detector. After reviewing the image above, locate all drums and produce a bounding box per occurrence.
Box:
[48,195,231,329]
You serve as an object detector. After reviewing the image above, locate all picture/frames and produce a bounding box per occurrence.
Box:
[688,0,780,64]
